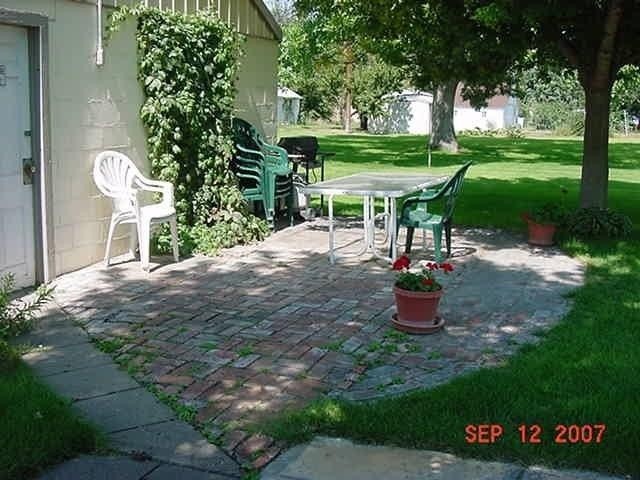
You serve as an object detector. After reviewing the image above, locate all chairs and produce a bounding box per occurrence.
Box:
[388,158,473,264]
[226,116,295,232]
[93,149,181,271]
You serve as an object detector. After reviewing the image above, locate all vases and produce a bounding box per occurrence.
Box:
[390,287,446,336]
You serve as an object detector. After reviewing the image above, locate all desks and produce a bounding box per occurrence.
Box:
[299,171,450,268]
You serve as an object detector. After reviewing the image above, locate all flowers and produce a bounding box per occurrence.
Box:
[392,256,454,292]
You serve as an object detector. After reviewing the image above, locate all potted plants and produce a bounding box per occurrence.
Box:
[521,204,563,248]
